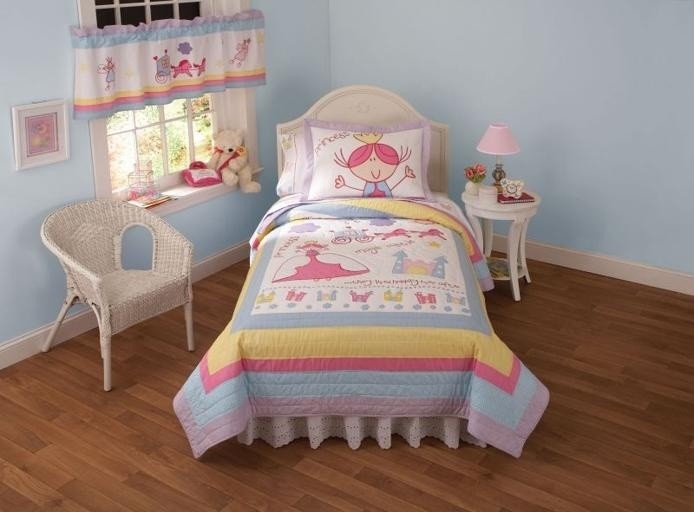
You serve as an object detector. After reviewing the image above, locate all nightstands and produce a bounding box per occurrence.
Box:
[460,182,541,303]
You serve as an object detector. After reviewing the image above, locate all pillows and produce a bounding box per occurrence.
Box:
[301,115,433,204]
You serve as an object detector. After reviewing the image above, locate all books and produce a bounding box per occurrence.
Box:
[497,191,535,204]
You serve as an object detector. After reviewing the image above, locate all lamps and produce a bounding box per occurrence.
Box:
[476,122,523,188]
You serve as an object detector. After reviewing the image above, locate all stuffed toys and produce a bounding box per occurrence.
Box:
[211,128,263,195]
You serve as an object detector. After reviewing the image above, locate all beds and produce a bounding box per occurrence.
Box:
[168,84,554,464]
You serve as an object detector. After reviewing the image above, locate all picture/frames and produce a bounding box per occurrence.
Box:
[9,98,71,174]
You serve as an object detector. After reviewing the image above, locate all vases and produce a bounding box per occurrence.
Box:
[462,180,480,194]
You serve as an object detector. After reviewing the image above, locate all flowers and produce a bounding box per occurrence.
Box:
[463,164,486,182]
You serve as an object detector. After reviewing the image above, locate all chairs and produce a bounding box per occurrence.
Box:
[40,198,194,392]
